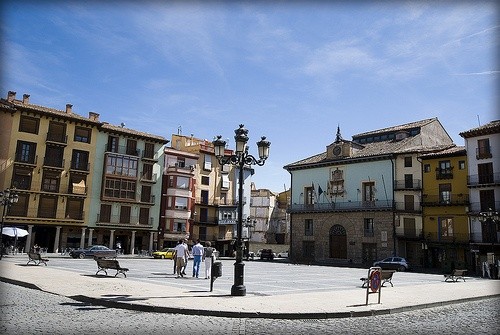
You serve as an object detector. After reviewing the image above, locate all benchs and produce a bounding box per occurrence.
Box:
[444,270,467,283]
[27,252,49,267]
[93,250,118,261]
[95,258,129,279]
[360,270,396,288]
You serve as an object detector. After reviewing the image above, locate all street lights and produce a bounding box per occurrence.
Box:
[0,185,20,247]
[211,123,272,297]
[242,217,256,238]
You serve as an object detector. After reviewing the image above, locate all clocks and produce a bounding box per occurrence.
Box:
[333,146,341,156]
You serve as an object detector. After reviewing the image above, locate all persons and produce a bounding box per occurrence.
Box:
[34,244,38,253]
[202,241,213,279]
[116,241,121,249]
[173,240,186,277]
[181,239,190,275]
[193,240,204,277]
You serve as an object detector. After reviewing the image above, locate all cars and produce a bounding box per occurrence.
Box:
[152,248,175,259]
[372,256,410,272]
[276,250,288,258]
[68,245,117,259]
[188,250,194,259]
[233,248,277,261]
